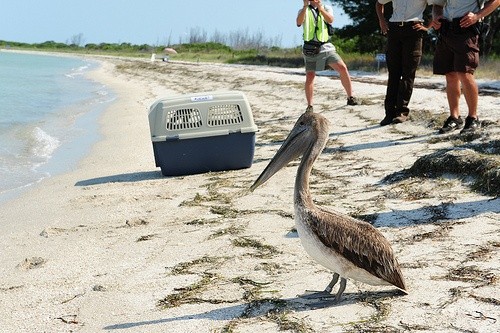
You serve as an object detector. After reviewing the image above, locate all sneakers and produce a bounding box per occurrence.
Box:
[460,115,481,135]
[438,115,464,134]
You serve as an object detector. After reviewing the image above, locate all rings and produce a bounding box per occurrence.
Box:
[466,22,468,25]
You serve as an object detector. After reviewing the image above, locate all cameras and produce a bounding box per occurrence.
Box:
[328,24,334,35]
[479,17,491,41]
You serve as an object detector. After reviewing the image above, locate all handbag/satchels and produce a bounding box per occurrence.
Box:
[327,24,333,35]
[444,17,481,43]
[303,43,320,57]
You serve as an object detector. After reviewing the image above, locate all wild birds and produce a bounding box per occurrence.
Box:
[249,111,407,308]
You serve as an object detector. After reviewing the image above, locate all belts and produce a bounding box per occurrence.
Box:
[390,21,415,26]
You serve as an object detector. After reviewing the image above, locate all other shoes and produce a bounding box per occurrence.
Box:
[379,113,408,127]
[347,96,362,106]
[305,106,313,113]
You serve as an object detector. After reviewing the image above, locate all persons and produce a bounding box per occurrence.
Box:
[432,0,500,135]
[376,0,434,125]
[296,0,360,114]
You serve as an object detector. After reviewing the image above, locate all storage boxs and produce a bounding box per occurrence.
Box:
[149,91,257,175]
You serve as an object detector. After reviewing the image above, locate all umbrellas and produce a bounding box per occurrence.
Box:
[163,47,176,54]
